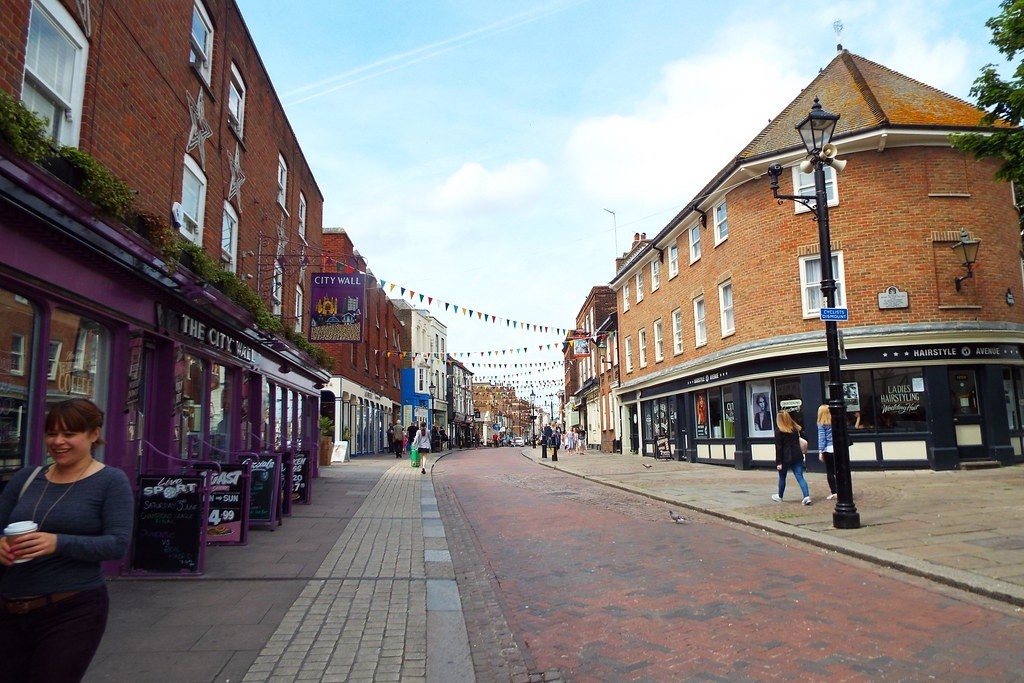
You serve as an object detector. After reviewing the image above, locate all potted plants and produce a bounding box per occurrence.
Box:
[318,416,333,467]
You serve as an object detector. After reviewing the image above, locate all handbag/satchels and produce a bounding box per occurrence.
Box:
[798,431,808,454]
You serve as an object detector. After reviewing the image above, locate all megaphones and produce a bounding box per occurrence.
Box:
[826,158,847,173]
[819,144,838,161]
[801,158,814,173]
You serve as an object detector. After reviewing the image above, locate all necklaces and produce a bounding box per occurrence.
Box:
[32,458,93,530]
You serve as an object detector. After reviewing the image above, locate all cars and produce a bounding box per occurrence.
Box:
[511,437,524,447]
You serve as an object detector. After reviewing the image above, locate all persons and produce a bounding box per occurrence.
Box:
[754,393,772,430]
[387,419,516,458]
[817,404,837,500]
[854,412,873,429]
[0,398,135,683]
[696,395,707,424]
[772,410,811,505]
[414,422,431,474]
[543,421,587,455]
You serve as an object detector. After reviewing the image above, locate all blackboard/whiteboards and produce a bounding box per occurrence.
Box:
[330,441,350,461]
[292,450,310,505]
[274,451,291,515]
[130,475,205,573]
[192,462,248,544]
[239,455,277,522]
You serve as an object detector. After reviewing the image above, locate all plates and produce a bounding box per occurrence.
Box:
[200,528,237,537]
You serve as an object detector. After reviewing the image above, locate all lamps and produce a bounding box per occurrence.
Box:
[951,227,981,292]
[313,378,325,390]
[170,281,216,306]
[278,358,292,373]
[259,339,290,351]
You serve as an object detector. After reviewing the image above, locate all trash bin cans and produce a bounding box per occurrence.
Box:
[432,435,441,452]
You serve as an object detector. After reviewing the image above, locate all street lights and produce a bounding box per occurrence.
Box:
[530,389,538,449]
[549,393,553,421]
[767,94,863,530]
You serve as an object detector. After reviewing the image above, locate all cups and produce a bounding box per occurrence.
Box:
[3,521,39,563]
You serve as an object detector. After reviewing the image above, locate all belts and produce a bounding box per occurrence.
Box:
[0,592,76,615]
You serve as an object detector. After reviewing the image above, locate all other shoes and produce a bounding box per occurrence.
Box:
[422,468,426,474]
[826,493,837,499]
[772,495,783,502]
[802,496,811,505]
